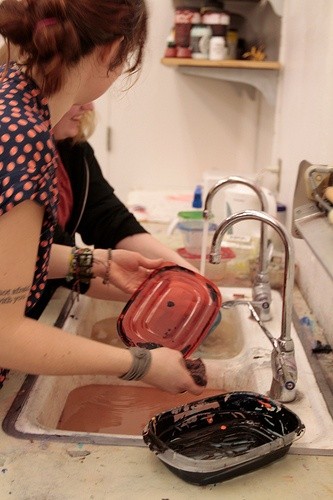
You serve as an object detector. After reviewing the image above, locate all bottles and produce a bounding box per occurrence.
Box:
[164,5,239,61]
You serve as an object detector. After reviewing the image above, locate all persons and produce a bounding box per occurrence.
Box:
[25,101,203,320]
[0,0,206,395]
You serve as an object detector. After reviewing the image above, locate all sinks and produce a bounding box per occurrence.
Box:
[71,291,245,361]
[32,363,260,440]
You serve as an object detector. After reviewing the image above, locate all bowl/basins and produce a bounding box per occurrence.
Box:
[173,210,236,279]
[142,390,306,485]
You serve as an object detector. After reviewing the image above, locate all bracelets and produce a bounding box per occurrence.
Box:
[118,346,151,381]
[66,247,96,302]
[103,249,112,285]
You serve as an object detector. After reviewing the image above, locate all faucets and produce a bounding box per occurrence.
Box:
[208,209,300,403]
[201,175,274,322]
